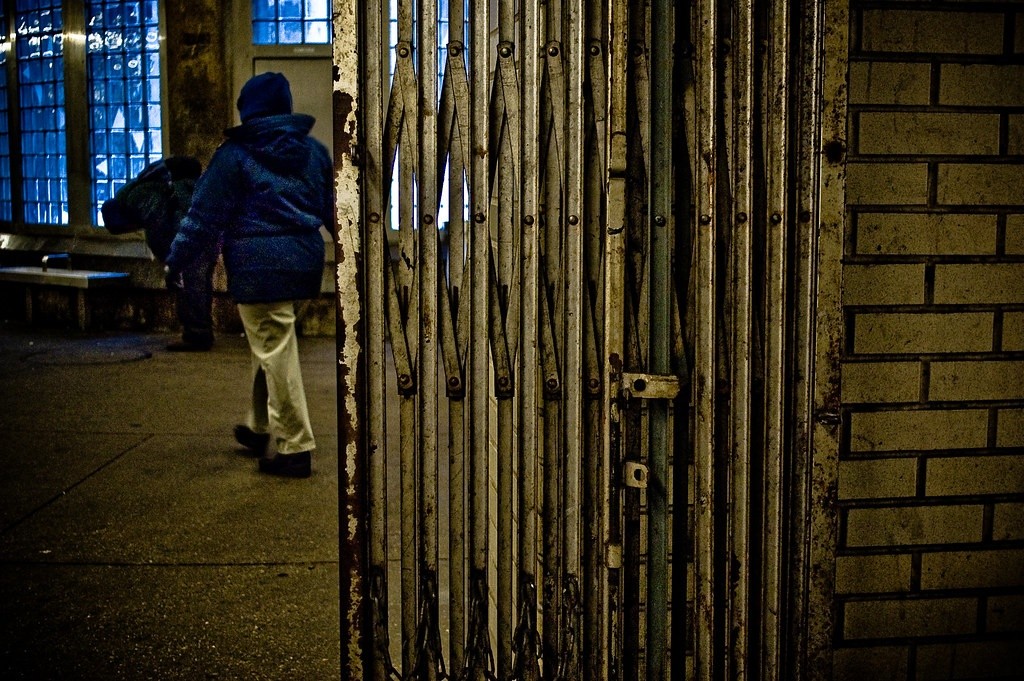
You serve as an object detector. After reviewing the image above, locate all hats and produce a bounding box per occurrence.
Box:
[100,198,135,229]
[236,71,293,124]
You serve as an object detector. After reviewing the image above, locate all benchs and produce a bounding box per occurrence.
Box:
[0,267,131,337]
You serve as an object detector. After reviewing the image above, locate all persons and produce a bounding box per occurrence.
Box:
[163,72,334,479]
[101,155,219,352]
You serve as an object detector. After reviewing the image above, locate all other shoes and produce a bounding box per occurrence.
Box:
[233,424,271,453]
[258,451,312,479]
[165,334,217,353]
